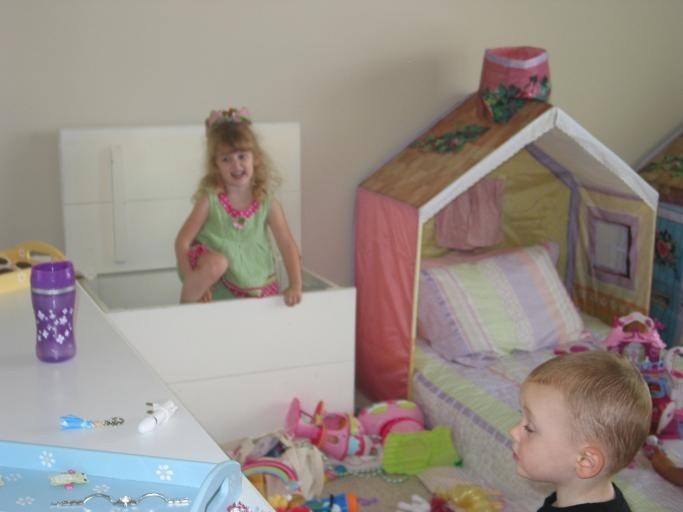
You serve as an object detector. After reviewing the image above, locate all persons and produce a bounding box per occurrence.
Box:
[510,350,652,511]
[172,108,303,306]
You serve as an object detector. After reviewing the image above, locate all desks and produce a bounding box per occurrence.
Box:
[1,249,273,512]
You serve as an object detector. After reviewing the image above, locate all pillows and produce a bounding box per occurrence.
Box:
[415,244,586,365]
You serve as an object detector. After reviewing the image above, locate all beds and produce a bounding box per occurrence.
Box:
[408,310,683,512]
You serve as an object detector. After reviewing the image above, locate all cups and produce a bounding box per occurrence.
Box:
[29,262,76,363]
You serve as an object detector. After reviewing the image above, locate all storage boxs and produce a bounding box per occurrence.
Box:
[59,122,359,449]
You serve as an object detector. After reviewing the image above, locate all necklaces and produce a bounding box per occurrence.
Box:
[218,191,257,228]
